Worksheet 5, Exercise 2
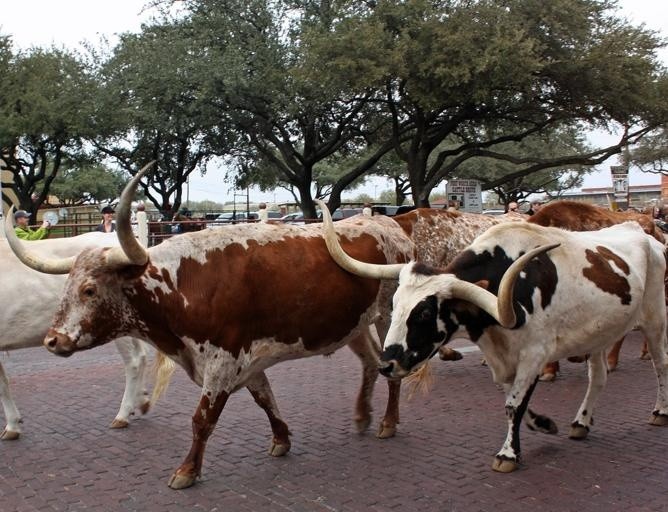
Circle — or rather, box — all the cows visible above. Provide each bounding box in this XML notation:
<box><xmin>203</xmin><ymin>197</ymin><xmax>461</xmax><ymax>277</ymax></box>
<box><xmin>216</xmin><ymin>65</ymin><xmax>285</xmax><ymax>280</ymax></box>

<box><xmin>0</xmin><ymin>231</ymin><xmax>179</xmax><ymax>441</ymax></box>
<box><xmin>311</xmin><ymin>198</ymin><xmax>668</xmax><ymax>474</ymax></box>
<box><xmin>390</xmin><ymin>207</ymin><xmax>530</xmax><ymax>361</ymax></box>
<box><xmin>527</xmin><ymin>201</ymin><xmax>665</xmax><ymax>382</ymax></box>
<box><xmin>5</xmin><ymin>160</ymin><xmax>432</xmax><ymax>490</ymax></box>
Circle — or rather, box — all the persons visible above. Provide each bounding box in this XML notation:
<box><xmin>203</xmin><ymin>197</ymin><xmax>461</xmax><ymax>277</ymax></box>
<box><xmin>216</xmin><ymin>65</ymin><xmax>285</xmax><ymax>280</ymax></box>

<box><xmin>507</xmin><ymin>201</ymin><xmax>519</xmax><ymax>213</ymax></box>
<box><xmin>653</xmin><ymin>204</ymin><xmax>668</xmax><ymax>235</ymax></box>
<box><xmin>93</xmin><ymin>206</ymin><xmax>117</xmax><ymax>233</ymax></box>
<box><xmin>524</xmin><ymin>201</ymin><xmax>541</xmax><ymax>216</ymax></box>
<box><xmin>13</xmin><ymin>210</ymin><xmax>51</xmax><ymax>241</ymax></box>
<box><xmin>173</xmin><ymin>207</ymin><xmax>190</xmax><ymax>226</ymax></box>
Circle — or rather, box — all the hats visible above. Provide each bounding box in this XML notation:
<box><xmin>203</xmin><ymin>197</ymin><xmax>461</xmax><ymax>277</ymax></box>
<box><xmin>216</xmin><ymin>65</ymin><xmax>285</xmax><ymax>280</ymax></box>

<box><xmin>531</xmin><ymin>199</ymin><xmax>544</xmax><ymax>205</ymax></box>
<box><xmin>101</xmin><ymin>207</ymin><xmax>115</xmax><ymax>214</ymax></box>
<box><xmin>510</xmin><ymin>202</ymin><xmax>517</xmax><ymax>209</ymax></box>
<box><xmin>14</xmin><ymin>210</ymin><xmax>32</xmax><ymax>218</ymax></box>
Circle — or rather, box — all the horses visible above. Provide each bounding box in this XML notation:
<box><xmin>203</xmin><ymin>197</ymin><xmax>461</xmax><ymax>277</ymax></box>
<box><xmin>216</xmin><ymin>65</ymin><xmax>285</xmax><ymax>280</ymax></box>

<box><xmin>170</xmin><ymin>212</ymin><xmax>206</xmax><ymax>233</ymax></box>
